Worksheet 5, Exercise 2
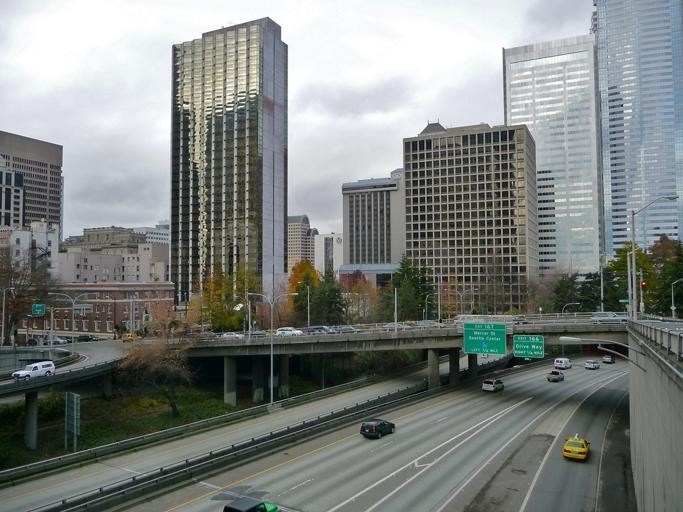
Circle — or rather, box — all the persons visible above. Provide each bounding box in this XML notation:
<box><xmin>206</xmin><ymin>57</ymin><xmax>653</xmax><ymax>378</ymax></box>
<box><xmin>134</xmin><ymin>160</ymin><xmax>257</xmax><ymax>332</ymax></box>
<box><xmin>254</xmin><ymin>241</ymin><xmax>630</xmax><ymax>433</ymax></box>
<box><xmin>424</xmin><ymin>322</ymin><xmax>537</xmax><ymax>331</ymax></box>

<box><xmin>439</xmin><ymin>318</ymin><xmax>442</xmax><ymax>323</ymax></box>
<box><xmin>113</xmin><ymin>332</ymin><xmax>116</xmax><ymax>340</ymax></box>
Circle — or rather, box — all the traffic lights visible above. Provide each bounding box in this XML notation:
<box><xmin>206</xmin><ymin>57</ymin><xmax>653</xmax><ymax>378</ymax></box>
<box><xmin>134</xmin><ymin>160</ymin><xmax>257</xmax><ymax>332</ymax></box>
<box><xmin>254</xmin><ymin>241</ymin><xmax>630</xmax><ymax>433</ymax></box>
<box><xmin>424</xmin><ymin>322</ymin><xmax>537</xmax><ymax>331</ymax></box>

<box><xmin>641</xmin><ymin>280</ymin><xmax>645</xmax><ymax>290</ymax></box>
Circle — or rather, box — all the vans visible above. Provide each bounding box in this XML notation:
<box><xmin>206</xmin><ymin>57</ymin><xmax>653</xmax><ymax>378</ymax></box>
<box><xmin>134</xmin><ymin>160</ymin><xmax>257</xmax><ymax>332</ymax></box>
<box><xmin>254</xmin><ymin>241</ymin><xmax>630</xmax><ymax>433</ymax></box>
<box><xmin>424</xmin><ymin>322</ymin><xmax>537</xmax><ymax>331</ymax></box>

<box><xmin>554</xmin><ymin>358</ymin><xmax>571</xmax><ymax>370</ymax></box>
<box><xmin>11</xmin><ymin>361</ymin><xmax>55</xmax><ymax>382</ymax></box>
<box><xmin>584</xmin><ymin>360</ymin><xmax>599</xmax><ymax>370</ymax></box>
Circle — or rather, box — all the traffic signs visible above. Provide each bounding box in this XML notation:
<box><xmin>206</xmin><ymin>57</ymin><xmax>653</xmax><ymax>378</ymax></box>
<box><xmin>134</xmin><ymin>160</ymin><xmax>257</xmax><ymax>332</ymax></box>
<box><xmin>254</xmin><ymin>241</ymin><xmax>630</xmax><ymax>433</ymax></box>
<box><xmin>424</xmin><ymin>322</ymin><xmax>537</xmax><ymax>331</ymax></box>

<box><xmin>31</xmin><ymin>304</ymin><xmax>45</xmax><ymax>315</ymax></box>
<box><xmin>618</xmin><ymin>299</ymin><xmax>628</xmax><ymax>303</ymax></box>
<box><xmin>462</xmin><ymin>322</ymin><xmax>506</xmax><ymax>356</ymax></box>
<box><xmin>512</xmin><ymin>335</ymin><xmax>544</xmax><ymax>359</ymax></box>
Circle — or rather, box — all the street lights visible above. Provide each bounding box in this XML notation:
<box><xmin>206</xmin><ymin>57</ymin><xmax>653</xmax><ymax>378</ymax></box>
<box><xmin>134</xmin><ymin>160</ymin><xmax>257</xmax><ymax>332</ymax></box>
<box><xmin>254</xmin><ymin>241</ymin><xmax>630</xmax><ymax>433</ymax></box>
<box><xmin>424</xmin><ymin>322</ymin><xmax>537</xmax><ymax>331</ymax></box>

<box><xmin>425</xmin><ymin>293</ymin><xmax>437</xmax><ymax>320</ymax></box>
<box><xmin>443</xmin><ymin>288</ymin><xmax>478</xmax><ymax>315</ymax></box>
<box><xmin>670</xmin><ymin>278</ymin><xmax>682</xmax><ymax>318</ymax></box>
<box><xmin>247</xmin><ymin>292</ymin><xmax>298</xmax><ymax>406</ymax></box>
<box><xmin>297</xmin><ymin>281</ymin><xmax>310</xmax><ymax>328</ymax></box>
<box><xmin>561</xmin><ymin>302</ymin><xmax>580</xmax><ymax>317</ymax></box>
<box><xmin>0</xmin><ymin>287</ymin><xmax>15</xmax><ymax>345</ymax></box>
<box><xmin>558</xmin><ymin>336</ymin><xmax>646</xmax><ymax>357</ymax></box>
<box><xmin>431</xmin><ymin>267</ymin><xmax>440</xmax><ymax>324</ymax></box>
<box><xmin>631</xmin><ymin>194</ymin><xmax>679</xmax><ymax>320</ymax></box>
<box><xmin>47</xmin><ymin>292</ymin><xmax>98</xmax><ymax>355</ymax></box>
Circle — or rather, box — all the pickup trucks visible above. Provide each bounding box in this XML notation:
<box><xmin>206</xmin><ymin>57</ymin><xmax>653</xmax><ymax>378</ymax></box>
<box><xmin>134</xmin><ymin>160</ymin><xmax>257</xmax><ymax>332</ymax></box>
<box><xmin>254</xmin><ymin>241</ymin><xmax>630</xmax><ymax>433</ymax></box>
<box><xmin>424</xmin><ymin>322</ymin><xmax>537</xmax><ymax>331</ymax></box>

<box><xmin>546</xmin><ymin>370</ymin><xmax>563</xmax><ymax>382</ymax></box>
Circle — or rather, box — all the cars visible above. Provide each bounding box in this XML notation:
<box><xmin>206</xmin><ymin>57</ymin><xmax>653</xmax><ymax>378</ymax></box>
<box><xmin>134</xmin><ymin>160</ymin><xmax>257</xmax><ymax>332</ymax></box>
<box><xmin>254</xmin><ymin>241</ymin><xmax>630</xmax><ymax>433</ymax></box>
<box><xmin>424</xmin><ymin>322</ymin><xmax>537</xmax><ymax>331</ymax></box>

<box><xmin>602</xmin><ymin>355</ymin><xmax>614</xmax><ymax>364</ymax></box>
<box><xmin>592</xmin><ymin>311</ymin><xmax>628</xmax><ymax>324</ymax></box>
<box><xmin>481</xmin><ymin>379</ymin><xmax>503</xmax><ymax>391</ymax></box>
<box><xmin>359</xmin><ymin>419</ymin><xmax>394</xmax><ymax>439</ymax></box>
<box><xmin>2</xmin><ymin>333</ymin><xmax>144</xmax><ymax>348</ymax></box>
<box><xmin>512</xmin><ymin>316</ymin><xmax>528</xmax><ymax>325</ymax></box>
<box><xmin>183</xmin><ymin>320</ymin><xmax>447</xmax><ymax>341</ymax></box>
<box><xmin>562</xmin><ymin>437</ymin><xmax>589</xmax><ymax>460</ymax></box>
